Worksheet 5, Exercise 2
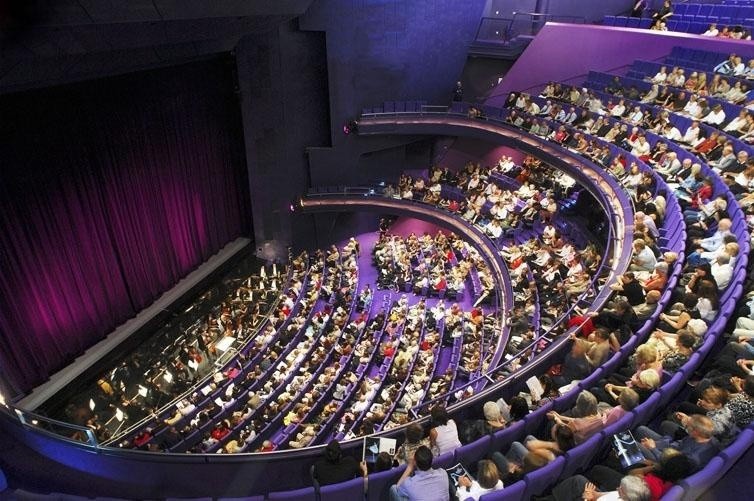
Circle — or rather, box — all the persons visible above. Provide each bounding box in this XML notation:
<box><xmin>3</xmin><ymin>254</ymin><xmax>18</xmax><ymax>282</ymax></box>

<box><xmin>651</xmin><ymin>1</ymin><xmax>673</xmax><ymax>21</ymax></box>
<box><xmin>60</xmin><ymin>53</ymin><xmax>753</xmax><ymax>501</ymax></box>
<box><xmin>701</xmin><ymin>23</ymin><xmax>752</xmax><ymax>40</ymax></box>
<box><xmin>651</xmin><ymin>21</ymin><xmax>669</xmax><ymax>31</ymax></box>
<box><xmin>629</xmin><ymin>0</ymin><xmax>648</xmax><ymax>18</ymax></box>
